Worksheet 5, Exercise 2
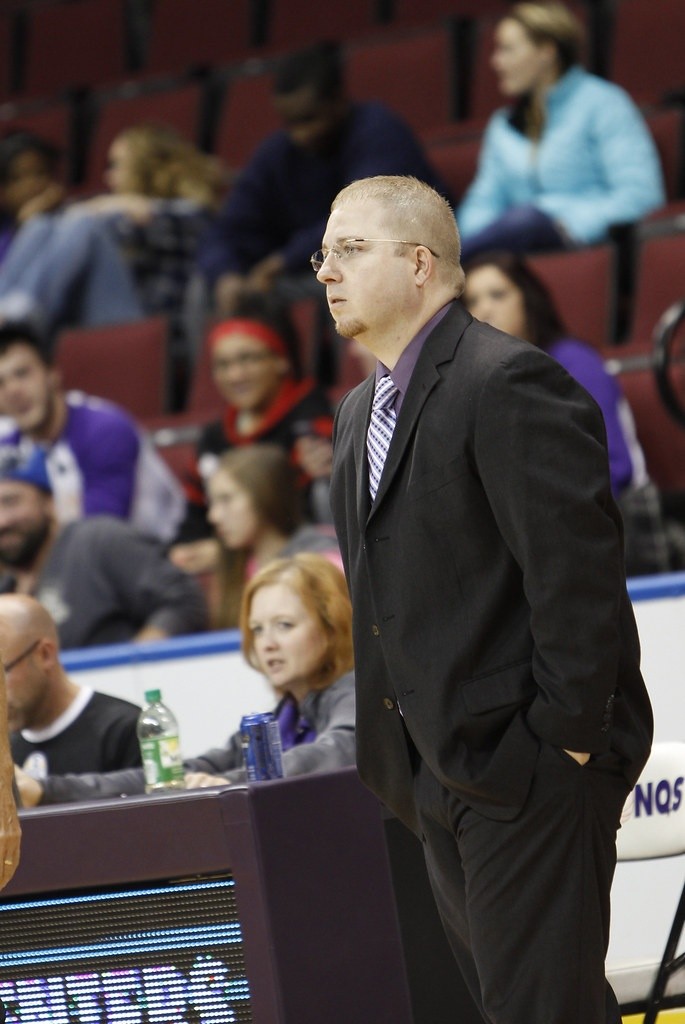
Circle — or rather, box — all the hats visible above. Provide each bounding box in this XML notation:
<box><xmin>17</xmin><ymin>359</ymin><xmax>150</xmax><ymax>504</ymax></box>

<box><xmin>0</xmin><ymin>443</ymin><xmax>53</xmax><ymax>495</ymax></box>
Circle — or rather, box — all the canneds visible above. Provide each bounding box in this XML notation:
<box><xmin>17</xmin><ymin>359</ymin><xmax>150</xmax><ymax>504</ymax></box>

<box><xmin>239</xmin><ymin>712</ymin><xmax>283</xmax><ymax>782</ymax></box>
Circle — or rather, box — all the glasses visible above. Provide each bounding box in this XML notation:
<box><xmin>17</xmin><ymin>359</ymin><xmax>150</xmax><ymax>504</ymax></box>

<box><xmin>310</xmin><ymin>238</ymin><xmax>439</xmax><ymax>272</ymax></box>
<box><xmin>279</xmin><ymin>103</ymin><xmax>329</xmax><ymax>130</ymax></box>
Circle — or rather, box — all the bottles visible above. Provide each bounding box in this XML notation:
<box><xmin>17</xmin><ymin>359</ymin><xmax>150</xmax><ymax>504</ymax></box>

<box><xmin>136</xmin><ymin>689</ymin><xmax>186</xmax><ymax>795</ymax></box>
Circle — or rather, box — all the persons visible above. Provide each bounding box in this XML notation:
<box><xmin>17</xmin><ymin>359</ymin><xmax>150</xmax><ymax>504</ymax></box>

<box><xmin>12</xmin><ymin>554</ymin><xmax>357</xmax><ymax>810</ymax></box>
<box><xmin>0</xmin><ymin>0</ymin><xmax>666</xmax><ymax>643</ymax></box>
<box><xmin>312</xmin><ymin>176</ymin><xmax>654</xmax><ymax>1024</ymax></box>
<box><xmin>0</xmin><ymin>589</ymin><xmax>160</xmax><ymax>808</ymax></box>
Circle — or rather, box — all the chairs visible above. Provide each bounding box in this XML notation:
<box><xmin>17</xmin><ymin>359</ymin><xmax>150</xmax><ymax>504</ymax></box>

<box><xmin>0</xmin><ymin>0</ymin><xmax>685</xmax><ymax>487</ymax></box>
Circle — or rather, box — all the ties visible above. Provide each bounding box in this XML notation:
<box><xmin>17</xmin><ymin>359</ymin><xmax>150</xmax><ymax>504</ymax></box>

<box><xmin>365</xmin><ymin>374</ymin><xmax>399</xmax><ymax>502</ymax></box>
